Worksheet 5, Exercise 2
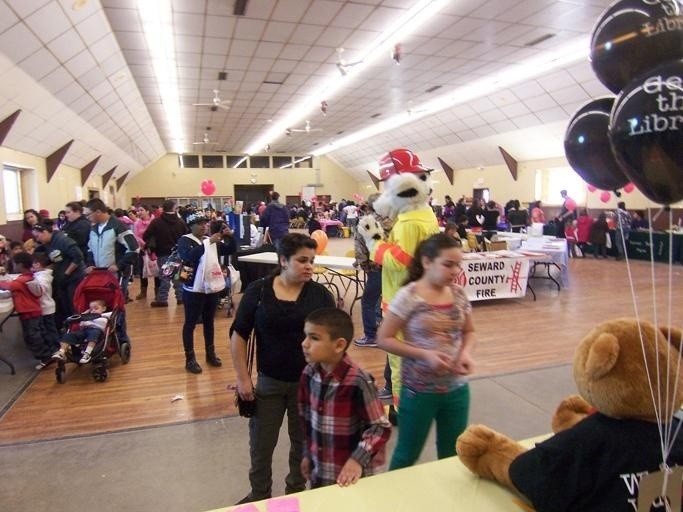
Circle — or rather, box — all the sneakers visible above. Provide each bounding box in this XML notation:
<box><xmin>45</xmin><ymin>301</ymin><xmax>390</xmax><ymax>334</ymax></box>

<box><xmin>567</xmin><ymin>252</ymin><xmax>625</xmax><ymax>262</ymax></box>
<box><xmin>352</xmin><ymin>332</ymin><xmax>380</xmax><ymax>348</ymax></box>
<box><xmin>376</xmin><ymin>386</ymin><xmax>394</xmax><ymax>401</ymax></box>
<box><xmin>34</xmin><ymin>360</ymin><xmax>51</xmax><ymax>370</ymax></box>
<box><xmin>77</xmin><ymin>352</ymin><xmax>92</xmax><ymax>364</ymax></box>
<box><xmin>150</xmin><ymin>298</ymin><xmax>185</xmax><ymax>308</ymax></box>
<box><xmin>51</xmin><ymin>350</ymin><xmax>68</xmax><ymax>362</ymax></box>
<box><xmin>233</xmin><ymin>494</ymin><xmax>274</xmax><ymax>507</ymax></box>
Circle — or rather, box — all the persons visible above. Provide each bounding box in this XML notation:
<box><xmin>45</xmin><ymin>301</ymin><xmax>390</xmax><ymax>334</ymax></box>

<box><xmin>229</xmin><ymin>234</ymin><xmax>336</xmax><ymax>506</ymax></box>
<box><xmin>429</xmin><ymin>189</ymin><xmax>651</xmax><ymax>260</ymax></box>
<box><xmin>296</xmin><ymin>306</ymin><xmax>392</xmax><ymax>490</ymax></box>
<box><xmin>375</xmin><ymin>233</ymin><xmax>475</xmax><ymax>472</ymax></box>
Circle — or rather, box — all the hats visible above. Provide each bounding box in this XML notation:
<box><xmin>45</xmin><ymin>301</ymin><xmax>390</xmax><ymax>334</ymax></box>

<box><xmin>184</xmin><ymin>206</ymin><xmax>211</xmax><ymax>225</ymax></box>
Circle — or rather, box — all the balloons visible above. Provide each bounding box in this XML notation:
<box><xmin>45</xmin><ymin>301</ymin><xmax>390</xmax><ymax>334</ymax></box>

<box><xmin>564</xmin><ymin>98</ymin><xmax>631</xmax><ymax>198</ymax></box>
<box><xmin>201</xmin><ymin>179</ymin><xmax>216</xmax><ymax>196</ymax></box>
<box><xmin>623</xmin><ymin>183</ymin><xmax>634</xmax><ymax>193</ymax></box>
<box><xmin>590</xmin><ymin>0</ymin><xmax>683</xmax><ymax>96</ymax></box>
<box><xmin>607</xmin><ymin>61</ymin><xmax>683</xmax><ymax>212</ymax></box>
<box><xmin>588</xmin><ymin>183</ymin><xmax>597</xmax><ymax>193</ymax></box>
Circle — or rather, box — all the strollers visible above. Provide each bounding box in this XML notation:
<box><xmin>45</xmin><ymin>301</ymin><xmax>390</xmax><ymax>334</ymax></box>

<box><xmin>53</xmin><ymin>265</ymin><xmax>131</xmax><ymax>388</ymax></box>
<box><xmin>213</xmin><ymin>254</ymin><xmax>236</xmax><ymax>318</ymax></box>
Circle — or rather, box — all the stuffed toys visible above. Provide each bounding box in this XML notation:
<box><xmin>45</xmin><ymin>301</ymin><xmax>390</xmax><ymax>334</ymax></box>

<box><xmin>455</xmin><ymin>317</ymin><xmax>683</xmax><ymax>512</ymax></box>
<box><xmin>355</xmin><ymin>172</ymin><xmax>439</xmax><ymax>425</ymax></box>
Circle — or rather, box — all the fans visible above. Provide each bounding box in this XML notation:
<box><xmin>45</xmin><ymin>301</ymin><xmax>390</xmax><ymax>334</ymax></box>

<box><xmin>193</xmin><ymin>48</ymin><xmax>427</xmax><ymax>145</ymax></box>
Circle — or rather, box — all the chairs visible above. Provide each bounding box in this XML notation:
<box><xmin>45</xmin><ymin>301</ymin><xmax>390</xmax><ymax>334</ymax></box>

<box><xmin>291</xmin><ymin>218</ymin><xmax>343</xmax><ymax>238</ymax></box>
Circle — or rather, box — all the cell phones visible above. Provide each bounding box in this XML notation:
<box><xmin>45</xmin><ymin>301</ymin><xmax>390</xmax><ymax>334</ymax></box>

<box><xmin>219</xmin><ymin>225</ymin><xmax>225</xmax><ymax>233</ymax></box>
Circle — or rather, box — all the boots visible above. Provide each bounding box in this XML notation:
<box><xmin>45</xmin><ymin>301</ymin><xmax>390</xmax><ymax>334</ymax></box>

<box><xmin>183</xmin><ymin>350</ymin><xmax>203</xmax><ymax>375</ymax></box>
<box><xmin>136</xmin><ymin>285</ymin><xmax>147</xmax><ymax>300</ymax></box>
<box><xmin>203</xmin><ymin>344</ymin><xmax>223</xmax><ymax>367</ymax></box>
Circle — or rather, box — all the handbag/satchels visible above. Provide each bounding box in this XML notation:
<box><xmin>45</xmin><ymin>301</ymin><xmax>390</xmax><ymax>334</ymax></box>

<box><xmin>234</xmin><ymin>385</ymin><xmax>257</xmax><ymax>420</ymax></box>
<box><xmin>159</xmin><ymin>235</ymin><xmax>196</xmax><ymax>283</ymax></box>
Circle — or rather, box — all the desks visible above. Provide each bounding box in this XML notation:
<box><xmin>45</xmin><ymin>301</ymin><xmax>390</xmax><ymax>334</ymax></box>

<box><xmin>438</xmin><ymin>219</ymin><xmax>569</xmax><ymax>302</ymax></box>
<box><xmin>237</xmin><ymin>251</ymin><xmax>368</xmax><ymax>316</ymax></box>
<box><xmin>205</xmin><ymin>432</ymin><xmax>555</xmax><ymax>512</ymax></box>
<box><xmin>0</xmin><ymin>272</ymin><xmax>32</xmax><ymax>376</ymax></box>
